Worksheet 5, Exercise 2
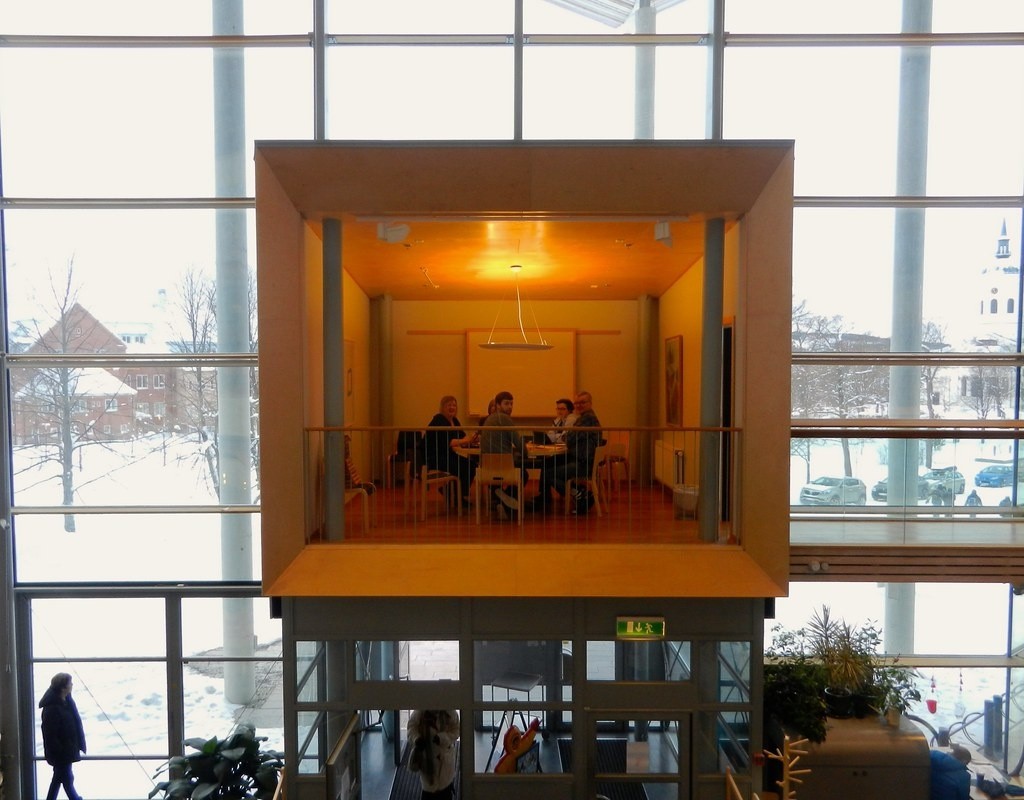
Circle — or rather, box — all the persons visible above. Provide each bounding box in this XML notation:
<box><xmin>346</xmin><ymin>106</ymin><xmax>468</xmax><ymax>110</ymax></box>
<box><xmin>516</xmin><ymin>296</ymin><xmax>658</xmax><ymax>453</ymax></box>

<box><xmin>420</xmin><ymin>396</ymin><xmax>480</xmax><ymax>510</ymax></box>
<box><xmin>38</xmin><ymin>673</ymin><xmax>86</xmax><ymax>800</ymax></box>
<box><xmin>932</xmin><ymin>486</ymin><xmax>1013</xmax><ymax>518</ymax></box>
<box><xmin>930</xmin><ymin>747</ymin><xmax>974</xmax><ymax>800</ymax></box>
<box><xmin>407</xmin><ymin>710</ymin><xmax>460</xmax><ymax>800</ymax></box>
<box><xmin>533</xmin><ymin>391</ymin><xmax>602</xmax><ymax>515</ymax></box>
<box><xmin>479</xmin><ymin>392</ymin><xmax>529</xmax><ymax>520</ymax></box>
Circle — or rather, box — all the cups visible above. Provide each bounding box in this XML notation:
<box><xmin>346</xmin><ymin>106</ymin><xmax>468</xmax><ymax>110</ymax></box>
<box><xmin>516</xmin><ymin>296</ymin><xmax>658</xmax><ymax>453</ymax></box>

<box><xmin>977</xmin><ymin>773</ymin><xmax>984</xmax><ymax>785</ymax></box>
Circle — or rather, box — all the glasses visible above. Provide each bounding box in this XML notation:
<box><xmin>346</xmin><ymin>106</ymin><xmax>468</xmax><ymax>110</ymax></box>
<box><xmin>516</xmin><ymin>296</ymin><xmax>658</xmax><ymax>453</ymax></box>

<box><xmin>574</xmin><ymin>400</ymin><xmax>590</xmax><ymax>404</ymax></box>
<box><xmin>556</xmin><ymin>408</ymin><xmax>567</xmax><ymax>411</ymax></box>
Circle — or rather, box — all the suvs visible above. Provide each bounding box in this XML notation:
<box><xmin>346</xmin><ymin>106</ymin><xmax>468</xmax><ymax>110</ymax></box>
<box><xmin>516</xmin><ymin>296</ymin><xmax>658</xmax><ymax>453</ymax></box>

<box><xmin>800</xmin><ymin>476</ymin><xmax>868</xmax><ymax>506</ymax></box>
<box><xmin>921</xmin><ymin>466</ymin><xmax>965</xmax><ymax>495</ymax></box>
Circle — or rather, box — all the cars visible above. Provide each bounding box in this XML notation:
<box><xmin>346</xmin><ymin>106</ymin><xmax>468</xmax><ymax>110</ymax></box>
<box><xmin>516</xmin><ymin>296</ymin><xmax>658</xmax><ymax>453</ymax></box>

<box><xmin>872</xmin><ymin>475</ymin><xmax>930</xmax><ymax>502</ymax></box>
<box><xmin>974</xmin><ymin>465</ymin><xmax>1014</xmax><ymax>488</ymax></box>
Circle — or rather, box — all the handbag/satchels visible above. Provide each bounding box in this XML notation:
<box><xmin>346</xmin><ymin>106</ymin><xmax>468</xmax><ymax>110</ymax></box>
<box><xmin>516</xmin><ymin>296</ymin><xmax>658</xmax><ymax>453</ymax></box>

<box><xmin>408</xmin><ymin>746</ymin><xmax>421</xmax><ymax>773</ymax></box>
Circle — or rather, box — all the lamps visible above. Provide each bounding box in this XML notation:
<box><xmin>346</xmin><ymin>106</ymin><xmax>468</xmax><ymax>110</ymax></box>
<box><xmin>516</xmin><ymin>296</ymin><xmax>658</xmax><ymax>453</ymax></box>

<box><xmin>654</xmin><ymin>223</ymin><xmax>674</xmax><ymax>248</ymax></box>
<box><xmin>376</xmin><ymin>220</ymin><xmax>408</xmax><ymax>243</ymax></box>
<box><xmin>478</xmin><ymin>264</ymin><xmax>554</xmax><ymax>351</ymax></box>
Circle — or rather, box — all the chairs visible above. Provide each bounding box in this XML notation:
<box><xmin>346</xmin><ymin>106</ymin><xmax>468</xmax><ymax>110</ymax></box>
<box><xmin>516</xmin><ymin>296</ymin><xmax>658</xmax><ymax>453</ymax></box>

<box><xmin>318</xmin><ymin>436</ymin><xmax>377</xmax><ymax>533</ymax></box>
<box><xmin>386</xmin><ymin>430</ymin><xmax>423</xmax><ymax>495</ymax></box>
<box><xmin>405</xmin><ymin>440</ymin><xmax>462</xmax><ymax>522</ymax></box>
<box><xmin>597</xmin><ymin>431</ymin><xmax>630</xmax><ymax>493</ymax></box>
<box><xmin>566</xmin><ymin>441</ymin><xmax>611</xmax><ymax>517</ymax></box>
<box><xmin>474</xmin><ymin>454</ymin><xmax>524</xmax><ymax>525</ymax></box>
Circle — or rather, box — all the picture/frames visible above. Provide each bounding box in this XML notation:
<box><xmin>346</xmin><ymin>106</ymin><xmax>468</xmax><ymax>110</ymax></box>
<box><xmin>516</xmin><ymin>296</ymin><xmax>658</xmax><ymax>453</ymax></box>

<box><xmin>663</xmin><ymin>335</ymin><xmax>682</xmax><ymax>425</ymax></box>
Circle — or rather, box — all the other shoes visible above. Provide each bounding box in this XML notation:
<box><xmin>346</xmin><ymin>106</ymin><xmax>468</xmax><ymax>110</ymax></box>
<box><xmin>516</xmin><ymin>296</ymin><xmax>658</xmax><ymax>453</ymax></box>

<box><xmin>494</xmin><ymin>488</ymin><xmax>518</xmax><ymax>520</ymax></box>
<box><xmin>575</xmin><ymin>483</ymin><xmax>595</xmax><ymax>515</ymax></box>
<box><xmin>530</xmin><ymin>493</ymin><xmax>553</xmax><ymax>513</ymax></box>
<box><xmin>438</xmin><ymin>484</ymin><xmax>472</xmax><ymax>509</ymax></box>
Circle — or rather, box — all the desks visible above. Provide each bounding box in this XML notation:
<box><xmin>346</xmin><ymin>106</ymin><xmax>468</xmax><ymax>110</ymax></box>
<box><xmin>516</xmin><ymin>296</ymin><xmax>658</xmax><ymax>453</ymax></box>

<box><xmin>490</xmin><ymin>672</ymin><xmax>544</xmax><ymax>746</ymax></box>
<box><xmin>787</xmin><ymin>710</ymin><xmax>930</xmax><ymax>800</ymax></box>
<box><xmin>451</xmin><ymin>439</ymin><xmax>569</xmax><ymax>459</ymax></box>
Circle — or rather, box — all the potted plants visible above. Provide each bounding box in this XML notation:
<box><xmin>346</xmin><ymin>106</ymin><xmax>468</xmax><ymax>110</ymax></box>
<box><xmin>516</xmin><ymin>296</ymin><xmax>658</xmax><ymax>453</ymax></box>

<box><xmin>768</xmin><ymin>610</ymin><xmax>920</xmax><ymax>730</ymax></box>
